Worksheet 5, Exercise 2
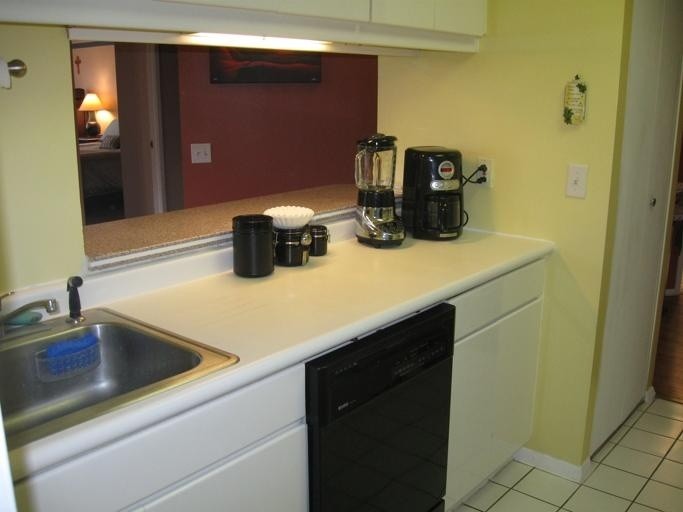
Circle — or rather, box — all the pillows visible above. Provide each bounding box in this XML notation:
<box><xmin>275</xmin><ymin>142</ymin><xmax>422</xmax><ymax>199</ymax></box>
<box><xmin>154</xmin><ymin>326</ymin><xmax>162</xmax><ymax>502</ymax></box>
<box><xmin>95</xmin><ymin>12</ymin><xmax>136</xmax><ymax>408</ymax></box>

<box><xmin>97</xmin><ymin>112</ymin><xmax>120</xmax><ymax>150</ymax></box>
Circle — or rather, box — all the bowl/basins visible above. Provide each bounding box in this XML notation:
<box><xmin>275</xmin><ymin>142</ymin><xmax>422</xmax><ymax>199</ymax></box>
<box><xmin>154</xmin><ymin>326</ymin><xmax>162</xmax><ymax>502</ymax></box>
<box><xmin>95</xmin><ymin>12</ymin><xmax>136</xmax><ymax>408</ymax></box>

<box><xmin>263</xmin><ymin>205</ymin><xmax>314</xmax><ymax>230</ymax></box>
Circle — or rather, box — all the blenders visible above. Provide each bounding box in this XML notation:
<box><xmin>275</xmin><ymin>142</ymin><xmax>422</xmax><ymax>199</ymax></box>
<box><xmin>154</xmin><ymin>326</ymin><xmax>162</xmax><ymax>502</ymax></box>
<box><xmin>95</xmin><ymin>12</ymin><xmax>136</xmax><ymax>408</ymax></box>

<box><xmin>354</xmin><ymin>133</ymin><xmax>405</xmax><ymax>247</ymax></box>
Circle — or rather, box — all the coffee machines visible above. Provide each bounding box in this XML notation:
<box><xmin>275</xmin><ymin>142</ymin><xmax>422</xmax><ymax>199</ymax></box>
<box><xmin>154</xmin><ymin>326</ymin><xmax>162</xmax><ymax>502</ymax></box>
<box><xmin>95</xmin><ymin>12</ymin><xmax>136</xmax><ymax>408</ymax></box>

<box><xmin>401</xmin><ymin>145</ymin><xmax>465</xmax><ymax>241</ymax></box>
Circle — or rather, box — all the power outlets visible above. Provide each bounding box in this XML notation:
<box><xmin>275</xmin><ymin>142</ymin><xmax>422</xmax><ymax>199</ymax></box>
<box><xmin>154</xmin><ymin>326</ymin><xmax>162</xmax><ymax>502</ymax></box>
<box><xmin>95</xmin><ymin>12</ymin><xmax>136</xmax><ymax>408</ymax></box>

<box><xmin>473</xmin><ymin>159</ymin><xmax>494</xmax><ymax>190</ymax></box>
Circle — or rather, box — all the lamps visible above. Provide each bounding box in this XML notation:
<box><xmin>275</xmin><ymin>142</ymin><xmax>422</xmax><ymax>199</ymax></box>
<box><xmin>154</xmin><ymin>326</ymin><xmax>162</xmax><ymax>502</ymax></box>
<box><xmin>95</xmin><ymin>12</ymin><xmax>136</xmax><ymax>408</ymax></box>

<box><xmin>78</xmin><ymin>94</ymin><xmax>101</xmax><ymax>139</ymax></box>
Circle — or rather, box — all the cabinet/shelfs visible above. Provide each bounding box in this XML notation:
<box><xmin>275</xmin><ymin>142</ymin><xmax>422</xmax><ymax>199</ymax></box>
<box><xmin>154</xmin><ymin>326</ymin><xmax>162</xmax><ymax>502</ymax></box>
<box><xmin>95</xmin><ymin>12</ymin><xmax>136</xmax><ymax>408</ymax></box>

<box><xmin>171</xmin><ymin>1</ymin><xmax>370</xmax><ymax>54</ymax></box>
<box><xmin>5</xmin><ymin>363</ymin><xmax>310</xmax><ymax>512</ymax></box>
<box><xmin>370</xmin><ymin>1</ymin><xmax>488</xmax><ymax>54</ymax></box>
<box><xmin>444</xmin><ymin>241</ymin><xmax>555</xmax><ymax>512</ymax></box>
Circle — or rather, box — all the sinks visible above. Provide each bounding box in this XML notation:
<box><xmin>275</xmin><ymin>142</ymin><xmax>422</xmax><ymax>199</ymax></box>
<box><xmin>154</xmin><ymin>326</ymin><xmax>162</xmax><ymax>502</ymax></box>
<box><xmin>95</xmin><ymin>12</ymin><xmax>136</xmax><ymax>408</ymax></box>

<box><xmin>3</xmin><ymin>306</ymin><xmax>240</xmax><ymax>452</ymax></box>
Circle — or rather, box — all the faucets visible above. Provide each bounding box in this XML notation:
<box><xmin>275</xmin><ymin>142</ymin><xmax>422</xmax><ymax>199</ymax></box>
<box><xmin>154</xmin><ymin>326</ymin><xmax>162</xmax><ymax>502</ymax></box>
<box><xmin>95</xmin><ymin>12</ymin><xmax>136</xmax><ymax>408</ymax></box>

<box><xmin>1</xmin><ymin>290</ymin><xmax>58</xmax><ymax>338</ymax></box>
